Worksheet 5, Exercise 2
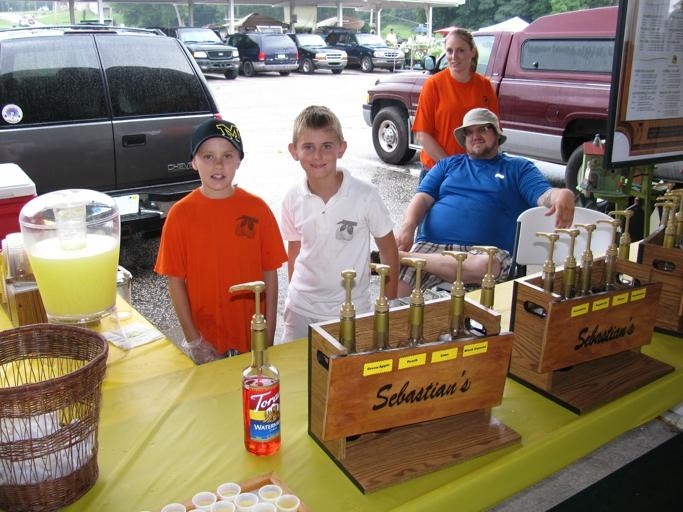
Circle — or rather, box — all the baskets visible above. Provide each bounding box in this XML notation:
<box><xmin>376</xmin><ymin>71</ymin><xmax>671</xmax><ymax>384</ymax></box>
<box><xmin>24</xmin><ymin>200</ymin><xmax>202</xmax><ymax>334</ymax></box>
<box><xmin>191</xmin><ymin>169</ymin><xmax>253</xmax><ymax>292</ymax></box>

<box><xmin>0</xmin><ymin>323</ymin><xmax>109</xmax><ymax>511</ymax></box>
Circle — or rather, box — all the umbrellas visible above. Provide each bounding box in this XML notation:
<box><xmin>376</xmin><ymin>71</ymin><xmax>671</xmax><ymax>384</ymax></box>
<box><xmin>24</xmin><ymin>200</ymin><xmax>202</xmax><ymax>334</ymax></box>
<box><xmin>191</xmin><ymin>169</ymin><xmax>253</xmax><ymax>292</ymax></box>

<box><xmin>409</xmin><ymin>26</ymin><xmax>428</xmax><ymax>32</ymax></box>
<box><xmin>434</xmin><ymin>27</ymin><xmax>459</xmax><ymax>34</ymax></box>
<box><xmin>477</xmin><ymin>16</ymin><xmax>531</xmax><ymax>32</ymax></box>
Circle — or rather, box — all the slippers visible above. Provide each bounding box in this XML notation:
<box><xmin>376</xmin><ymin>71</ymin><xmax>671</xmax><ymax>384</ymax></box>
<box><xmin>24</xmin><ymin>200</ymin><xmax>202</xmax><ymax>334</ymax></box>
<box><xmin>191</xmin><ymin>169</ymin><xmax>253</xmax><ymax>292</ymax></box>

<box><xmin>371</xmin><ymin>251</ymin><xmax>381</xmax><ymax>274</ymax></box>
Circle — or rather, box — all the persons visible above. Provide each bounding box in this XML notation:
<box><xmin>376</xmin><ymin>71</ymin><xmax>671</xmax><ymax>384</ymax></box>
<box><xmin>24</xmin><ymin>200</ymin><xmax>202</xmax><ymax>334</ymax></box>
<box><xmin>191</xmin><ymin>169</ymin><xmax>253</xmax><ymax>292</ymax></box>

<box><xmin>153</xmin><ymin>118</ymin><xmax>290</xmax><ymax>366</ymax></box>
<box><xmin>282</xmin><ymin>105</ymin><xmax>400</xmax><ymax>344</ymax></box>
<box><xmin>371</xmin><ymin>107</ymin><xmax>575</xmax><ymax>308</ymax></box>
<box><xmin>385</xmin><ymin>27</ymin><xmax>400</xmax><ymax>49</ymax></box>
<box><xmin>398</xmin><ymin>32</ymin><xmax>434</xmax><ymax>54</ymax></box>
<box><xmin>419</xmin><ymin>30</ymin><xmax>503</xmax><ymax>195</ymax></box>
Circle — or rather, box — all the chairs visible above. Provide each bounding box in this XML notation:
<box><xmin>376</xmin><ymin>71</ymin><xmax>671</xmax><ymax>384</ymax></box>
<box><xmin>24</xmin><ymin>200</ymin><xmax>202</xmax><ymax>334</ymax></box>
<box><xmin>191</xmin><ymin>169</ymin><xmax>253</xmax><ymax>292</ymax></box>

<box><xmin>506</xmin><ymin>205</ymin><xmax>621</xmax><ymax>280</ymax></box>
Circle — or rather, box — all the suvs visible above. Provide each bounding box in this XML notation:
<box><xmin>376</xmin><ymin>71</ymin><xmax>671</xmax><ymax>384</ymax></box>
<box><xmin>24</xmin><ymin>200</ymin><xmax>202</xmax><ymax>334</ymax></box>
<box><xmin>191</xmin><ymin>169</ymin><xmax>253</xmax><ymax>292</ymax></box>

<box><xmin>290</xmin><ymin>34</ymin><xmax>346</xmax><ymax>74</ymax></box>
<box><xmin>227</xmin><ymin>33</ymin><xmax>299</xmax><ymax>77</ymax></box>
<box><xmin>326</xmin><ymin>33</ymin><xmax>406</xmax><ymax>73</ymax></box>
<box><xmin>0</xmin><ymin>26</ymin><xmax>222</xmax><ymax>238</ymax></box>
<box><xmin>156</xmin><ymin>27</ymin><xmax>240</xmax><ymax>79</ymax></box>
<box><xmin>363</xmin><ymin>5</ymin><xmax>682</xmax><ymax>213</ymax></box>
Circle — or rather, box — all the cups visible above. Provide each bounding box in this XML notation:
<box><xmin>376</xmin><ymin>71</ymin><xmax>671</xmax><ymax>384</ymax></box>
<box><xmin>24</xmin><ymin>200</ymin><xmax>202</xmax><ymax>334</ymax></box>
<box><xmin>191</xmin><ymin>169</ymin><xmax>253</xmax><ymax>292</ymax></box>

<box><xmin>235</xmin><ymin>493</ymin><xmax>259</xmax><ymax>512</ymax></box>
<box><xmin>190</xmin><ymin>509</ymin><xmax>207</xmax><ymax>512</ymax></box>
<box><xmin>275</xmin><ymin>495</ymin><xmax>301</xmax><ymax>512</ymax></box>
<box><xmin>258</xmin><ymin>485</ymin><xmax>282</xmax><ymax>502</ymax></box>
<box><xmin>252</xmin><ymin>503</ymin><xmax>277</xmax><ymax>512</ymax></box>
<box><xmin>217</xmin><ymin>483</ymin><xmax>242</xmax><ymax>500</ymax></box>
<box><xmin>192</xmin><ymin>492</ymin><xmax>217</xmax><ymax>508</ymax></box>
<box><xmin>160</xmin><ymin>501</ymin><xmax>188</xmax><ymax>512</ymax></box>
<box><xmin>210</xmin><ymin>501</ymin><xmax>235</xmax><ymax>512</ymax></box>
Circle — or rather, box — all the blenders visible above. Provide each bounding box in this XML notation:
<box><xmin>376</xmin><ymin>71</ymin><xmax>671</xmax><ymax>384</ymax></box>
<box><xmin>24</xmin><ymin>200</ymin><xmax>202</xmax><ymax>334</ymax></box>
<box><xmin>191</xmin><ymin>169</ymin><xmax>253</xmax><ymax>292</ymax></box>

<box><xmin>19</xmin><ymin>189</ymin><xmax>131</xmax><ymax>366</ymax></box>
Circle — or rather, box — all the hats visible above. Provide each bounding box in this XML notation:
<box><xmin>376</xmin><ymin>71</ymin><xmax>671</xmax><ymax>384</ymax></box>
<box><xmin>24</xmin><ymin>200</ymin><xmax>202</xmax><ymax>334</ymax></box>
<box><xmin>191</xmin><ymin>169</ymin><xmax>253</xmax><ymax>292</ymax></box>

<box><xmin>192</xmin><ymin>119</ymin><xmax>244</xmax><ymax>160</ymax></box>
<box><xmin>454</xmin><ymin>108</ymin><xmax>506</xmax><ymax>148</ymax></box>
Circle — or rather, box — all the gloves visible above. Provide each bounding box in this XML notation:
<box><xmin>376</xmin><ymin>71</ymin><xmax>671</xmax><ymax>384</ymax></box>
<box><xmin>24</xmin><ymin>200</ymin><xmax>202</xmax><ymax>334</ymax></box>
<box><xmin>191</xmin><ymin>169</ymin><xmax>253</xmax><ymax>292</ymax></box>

<box><xmin>181</xmin><ymin>336</ymin><xmax>220</xmax><ymax>364</ymax></box>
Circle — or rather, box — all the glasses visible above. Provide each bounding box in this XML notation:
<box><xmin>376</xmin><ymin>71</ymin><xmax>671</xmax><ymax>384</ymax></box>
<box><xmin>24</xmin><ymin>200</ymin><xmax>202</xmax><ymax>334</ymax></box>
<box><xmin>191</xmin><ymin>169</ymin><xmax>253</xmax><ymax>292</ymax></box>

<box><xmin>462</xmin><ymin>125</ymin><xmax>494</xmax><ymax>136</ymax></box>
